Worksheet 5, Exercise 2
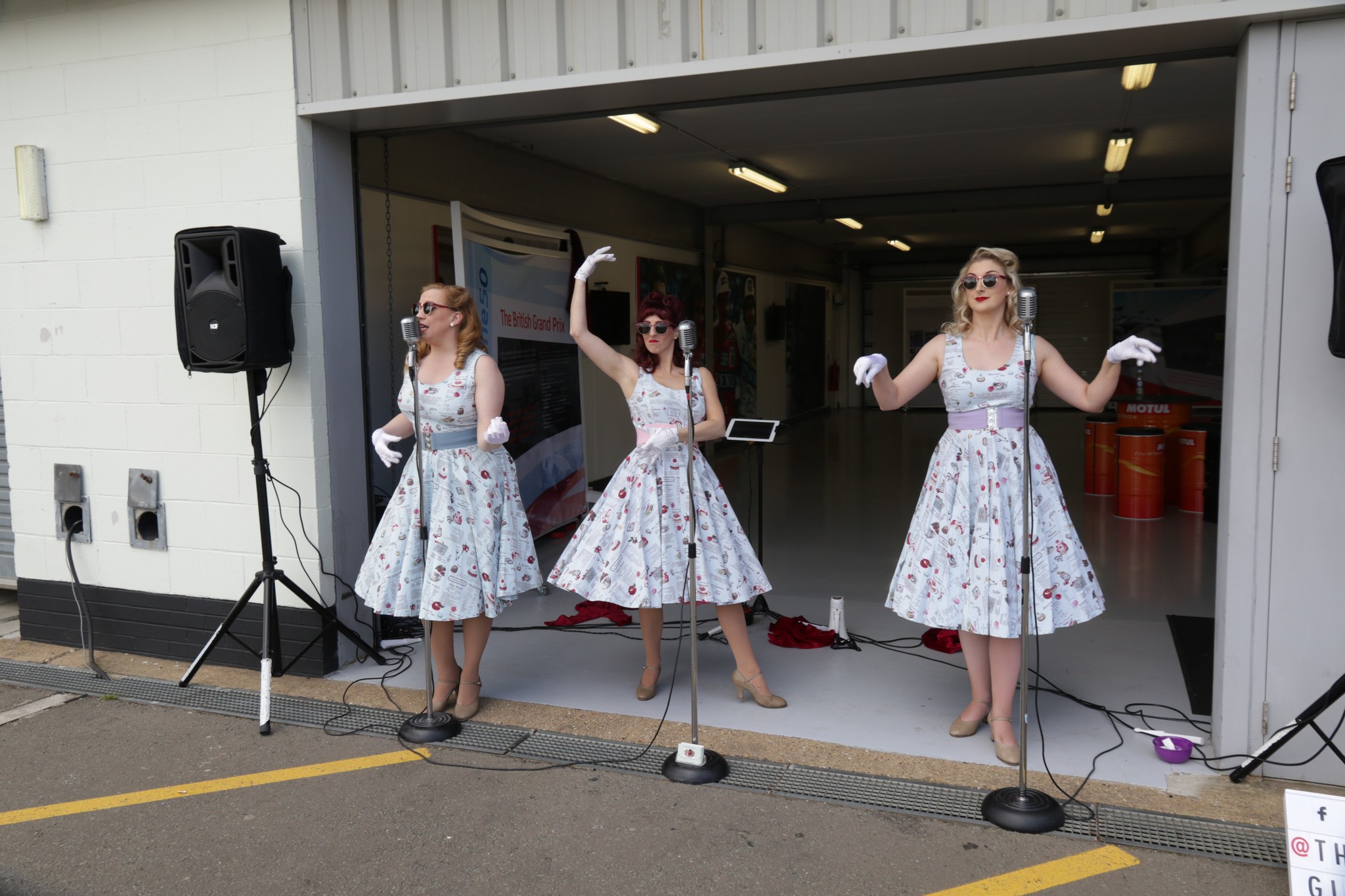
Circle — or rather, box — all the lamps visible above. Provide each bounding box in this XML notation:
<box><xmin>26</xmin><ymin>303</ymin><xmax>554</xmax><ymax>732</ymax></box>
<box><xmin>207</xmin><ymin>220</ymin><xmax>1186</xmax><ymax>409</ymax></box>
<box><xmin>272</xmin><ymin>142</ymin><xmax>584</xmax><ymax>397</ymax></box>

<box><xmin>16</xmin><ymin>145</ymin><xmax>50</xmax><ymax>223</ymax></box>
<box><xmin>728</xmin><ymin>161</ymin><xmax>790</xmax><ymax>193</ymax></box>
<box><xmin>606</xmin><ymin>113</ymin><xmax>661</xmax><ymax>135</ymax></box>
<box><xmin>1121</xmin><ymin>63</ymin><xmax>1157</xmax><ymax>90</ymax></box>
<box><xmin>1103</xmin><ymin>129</ymin><xmax>1134</xmax><ymax>172</ymax></box>
<box><xmin>835</xmin><ymin>218</ymin><xmax>911</xmax><ymax>252</ymax></box>
<box><xmin>1090</xmin><ymin>204</ymin><xmax>1113</xmax><ymax>244</ymax></box>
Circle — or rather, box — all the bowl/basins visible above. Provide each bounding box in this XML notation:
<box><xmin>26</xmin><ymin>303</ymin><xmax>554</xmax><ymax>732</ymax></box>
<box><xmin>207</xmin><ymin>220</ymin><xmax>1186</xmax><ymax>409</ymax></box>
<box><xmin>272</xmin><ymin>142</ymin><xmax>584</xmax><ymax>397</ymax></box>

<box><xmin>1152</xmin><ymin>735</ymin><xmax>1192</xmax><ymax>764</ymax></box>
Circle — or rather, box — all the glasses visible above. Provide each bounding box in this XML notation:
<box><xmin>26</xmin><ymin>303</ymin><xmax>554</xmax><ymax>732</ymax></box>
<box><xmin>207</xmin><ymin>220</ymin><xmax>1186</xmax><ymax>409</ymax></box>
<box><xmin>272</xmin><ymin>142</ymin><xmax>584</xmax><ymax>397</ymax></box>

<box><xmin>636</xmin><ymin>321</ymin><xmax>676</xmax><ymax>335</ymax></box>
<box><xmin>961</xmin><ymin>273</ymin><xmax>1008</xmax><ymax>290</ymax></box>
<box><xmin>412</xmin><ymin>302</ymin><xmax>459</xmax><ymax>315</ymax></box>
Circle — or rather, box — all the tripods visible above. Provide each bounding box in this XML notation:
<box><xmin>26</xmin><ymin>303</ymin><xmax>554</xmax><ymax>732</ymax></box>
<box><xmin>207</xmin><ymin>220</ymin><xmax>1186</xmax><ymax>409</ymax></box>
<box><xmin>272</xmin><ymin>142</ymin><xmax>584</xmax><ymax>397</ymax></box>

<box><xmin>176</xmin><ymin>370</ymin><xmax>385</xmax><ymax>736</ymax></box>
<box><xmin>698</xmin><ymin>443</ymin><xmax>789</xmax><ymax>640</ymax></box>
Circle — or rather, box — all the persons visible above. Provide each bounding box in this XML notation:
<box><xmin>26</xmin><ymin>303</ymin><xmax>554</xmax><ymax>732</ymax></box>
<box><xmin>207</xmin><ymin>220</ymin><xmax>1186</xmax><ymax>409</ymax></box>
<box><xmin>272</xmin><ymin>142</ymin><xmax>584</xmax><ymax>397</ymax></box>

<box><xmin>546</xmin><ymin>247</ymin><xmax>788</xmax><ymax>708</ymax></box>
<box><xmin>854</xmin><ymin>247</ymin><xmax>1161</xmax><ymax>767</ymax></box>
<box><xmin>353</xmin><ymin>285</ymin><xmax>543</xmax><ymax>722</ymax></box>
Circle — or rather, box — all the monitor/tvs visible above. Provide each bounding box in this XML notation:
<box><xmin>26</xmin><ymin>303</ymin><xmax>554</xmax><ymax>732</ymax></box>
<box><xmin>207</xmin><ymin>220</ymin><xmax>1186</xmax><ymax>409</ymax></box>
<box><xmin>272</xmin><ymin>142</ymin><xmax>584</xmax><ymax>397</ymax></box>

<box><xmin>590</xmin><ymin>290</ymin><xmax>631</xmax><ymax>346</ymax></box>
<box><xmin>764</xmin><ymin>305</ymin><xmax>786</xmax><ymax>341</ymax></box>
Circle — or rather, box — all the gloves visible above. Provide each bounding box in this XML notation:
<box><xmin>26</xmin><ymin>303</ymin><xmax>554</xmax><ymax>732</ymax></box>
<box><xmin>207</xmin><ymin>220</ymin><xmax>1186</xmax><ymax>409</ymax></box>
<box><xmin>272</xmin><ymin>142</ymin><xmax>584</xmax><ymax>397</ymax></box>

<box><xmin>636</xmin><ymin>425</ymin><xmax>679</xmax><ymax>466</ymax></box>
<box><xmin>574</xmin><ymin>246</ymin><xmax>616</xmax><ymax>282</ymax></box>
<box><xmin>484</xmin><ymin>416</ymin><xmax>510</xmax><ymax>444</ymax></box>
<box><xmin>372</xmin><ymin>429</ymin><xmax>402</xmax><ymax>468</ymax></box>
<box><xmin>1107</xmin><ymin>335</ymin><xmax>1162</xmax><ymax>366</ymax></box>
<box><xmin>853</xmin><ymin>353</ymin><xmax>887</xmax><ymax>388</ymax></box>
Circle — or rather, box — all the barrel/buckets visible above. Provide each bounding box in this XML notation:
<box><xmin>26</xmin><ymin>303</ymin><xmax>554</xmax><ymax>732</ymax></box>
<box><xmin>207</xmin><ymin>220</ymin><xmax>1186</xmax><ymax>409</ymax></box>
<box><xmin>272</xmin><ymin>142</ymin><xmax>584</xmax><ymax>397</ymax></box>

<box><xmin>1085</xmin><ymin>415</ymin><xmax>1117</xmax><ymax>497</ymax></box>
<box><xmin>1113</xmin><ymin>426</ymin><xmax>1164</xmax><ymax>521</ymax></box>
<box><xmin>1177</xmin><ymin>424</ymin><xmax>1208</xmax><ymax>514</ymax></box>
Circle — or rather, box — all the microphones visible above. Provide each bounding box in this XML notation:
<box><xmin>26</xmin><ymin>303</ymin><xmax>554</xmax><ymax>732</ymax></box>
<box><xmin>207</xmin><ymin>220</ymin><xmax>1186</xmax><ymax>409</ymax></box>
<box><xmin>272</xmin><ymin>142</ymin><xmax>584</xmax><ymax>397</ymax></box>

<box><xmin>676</xmin><ymin>320</ymin><xmax>697</xmax><ymax>350</ymax></box>
<box><xmin>1017</xmin><ymin>287</ymin><xmax>1037</xmax><ymax>321</ymax></box>
<box><xmin>400</xmin><ymin>316</ymin><xmax>421</xmax><ymax>343</ymax></box>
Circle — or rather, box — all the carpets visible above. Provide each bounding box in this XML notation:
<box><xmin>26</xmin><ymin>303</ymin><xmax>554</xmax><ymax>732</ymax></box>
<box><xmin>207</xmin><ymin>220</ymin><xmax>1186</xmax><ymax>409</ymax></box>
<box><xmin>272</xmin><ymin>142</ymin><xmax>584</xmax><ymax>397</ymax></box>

<box><xmin>1167</xmin><ymin>613</ymin><xmax>1214</xmax><ymax>716</ymax></box>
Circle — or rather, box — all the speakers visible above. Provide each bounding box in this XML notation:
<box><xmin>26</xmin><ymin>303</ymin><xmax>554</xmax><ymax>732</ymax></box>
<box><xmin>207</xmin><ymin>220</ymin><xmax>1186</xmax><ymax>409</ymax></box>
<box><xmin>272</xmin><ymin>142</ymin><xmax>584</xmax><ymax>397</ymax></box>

<box><xmin>1315</xmin><ymin>156</ymin><xmax>1345</xmax><ymax>359</ymax></box>
<box><xmin>174</xmin><ymin>225</ymin><xmax>294</xmax><ymax>374</ymax></box>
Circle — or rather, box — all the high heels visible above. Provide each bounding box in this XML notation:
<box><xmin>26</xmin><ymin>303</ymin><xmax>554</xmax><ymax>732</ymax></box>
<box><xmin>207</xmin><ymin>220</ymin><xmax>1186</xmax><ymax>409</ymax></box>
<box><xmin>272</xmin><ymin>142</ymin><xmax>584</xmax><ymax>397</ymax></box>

<box><xmin>731</xmin><ymin>669</ymin><xmax>787</xmax><ymax>709</ymax></box>
<box><xmin>454</xmin><ymin>675</ymin><xmax>482</xmax><ymax>721</ymax></box>
<box><xmin>432</xmin><ymin>666</ymin><xmax>462</xmax><ymax>713</ymax></box>
<box><xmin>950</xmin><ymin>697</ymin><xmax>992</xmax><ymax>737</ymax></box>
<box><xmin>637</xmin><ymin>667</ymin><xmax>662</xmax><ymax>701</ymax></box>
<box><xmin>988</xmin><ymin>709</ymin><xmax>1021</xmax><ymax>766</ymax></box>
